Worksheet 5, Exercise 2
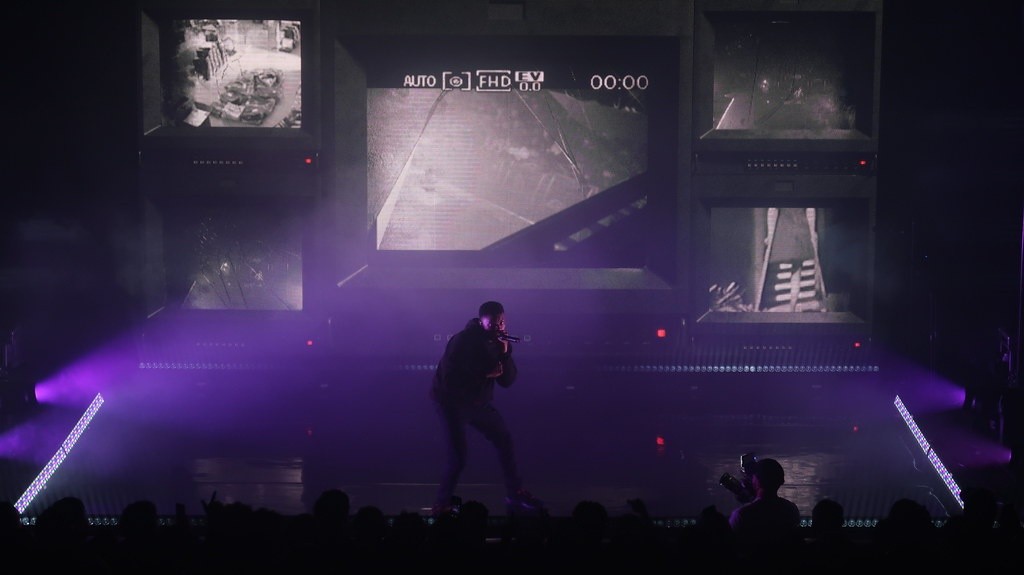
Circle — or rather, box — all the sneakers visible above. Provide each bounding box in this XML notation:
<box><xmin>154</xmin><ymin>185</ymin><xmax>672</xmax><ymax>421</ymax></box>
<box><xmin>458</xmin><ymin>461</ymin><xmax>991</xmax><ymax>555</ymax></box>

<box><xmin>502</xmin><ymin>487</ymin><xmax>538</xmax><ymax>511</ymax></box>
<box><xmin>442</xmin><ymin>501</ymin><xmax>463</xmax><ymax>519</ymax></box>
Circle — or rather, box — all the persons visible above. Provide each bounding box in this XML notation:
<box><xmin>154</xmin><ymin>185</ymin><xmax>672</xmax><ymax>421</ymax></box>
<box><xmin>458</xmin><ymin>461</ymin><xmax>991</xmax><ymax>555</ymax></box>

<box><xmin>0</xmin><ymin>458</ymin><xmax>1024</xmax><ymax>575</ymax></box>
<box><xmin>429</xmin><ymin>301</ymin><xmax>545</xmax><ymax>510</ymax></box>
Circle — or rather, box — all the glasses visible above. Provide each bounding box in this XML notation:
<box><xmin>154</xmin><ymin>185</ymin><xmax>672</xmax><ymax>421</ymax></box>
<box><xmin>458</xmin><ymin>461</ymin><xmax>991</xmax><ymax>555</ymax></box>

<box><xmin>482</xmin><ymin>321</ymin><xmax>505</xmax><ymax>327</ymax></box>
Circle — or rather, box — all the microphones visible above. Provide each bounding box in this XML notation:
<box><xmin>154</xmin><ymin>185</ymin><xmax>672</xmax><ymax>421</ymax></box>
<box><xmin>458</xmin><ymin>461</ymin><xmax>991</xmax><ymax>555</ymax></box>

<box><xmin>498</xmin><ymin>334</ymin><xmax>520</xmax><ymax>343</ymax></box>
